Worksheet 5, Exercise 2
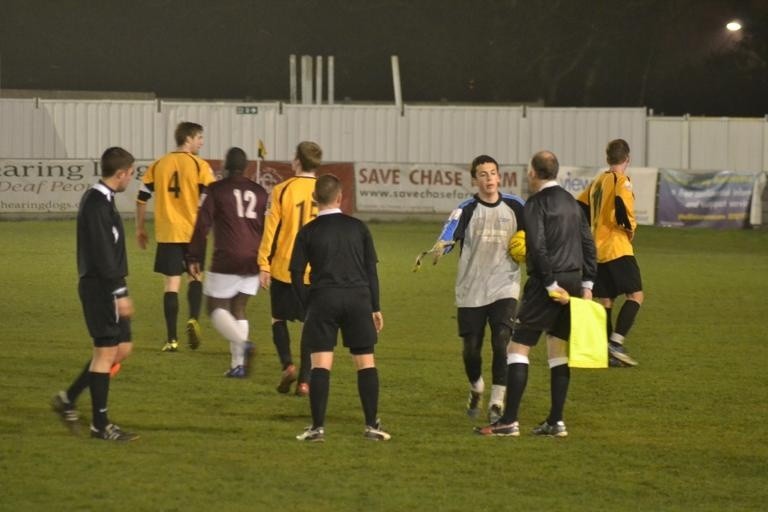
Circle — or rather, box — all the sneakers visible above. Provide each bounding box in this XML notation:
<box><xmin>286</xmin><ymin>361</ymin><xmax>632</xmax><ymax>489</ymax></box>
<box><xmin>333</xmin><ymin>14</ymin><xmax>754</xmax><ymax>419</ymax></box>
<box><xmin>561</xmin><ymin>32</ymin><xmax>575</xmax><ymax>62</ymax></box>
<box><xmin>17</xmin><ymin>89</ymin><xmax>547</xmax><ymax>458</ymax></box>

<box><xmin>52</xmin><ymin>391</ymin><xmax>82</xmax><ymax>437</ymax></box>
<box><xmin>362</xmin><ymin>426</ymin><xmax>392</xmax><ymax>443</ymax></box>
<box><xmin>607</xmin><ymin>338</ymin><xmax>638</xmax><ymax>368</ymax></box>
<box><xmin>294</xmin><ymin>426</ymin><xmax>324</xmax><ymax>443</ymax></box>
<box><xmin>91</xmin><ymin>425</ymin><xmax>141</xmax><ymax>442</ymax></box>
<box><xmin>294</xmin><ymin>384</ymin><xmax>309</xmax><ymax>396</ymax></box>
<box><xmin>162</xmin><ymin>341</ymin><xmax>178</xmax><ymax>351</ymax></box>
<box><xmin>224</xmin><ymin>341</ymin><xmax>254</xmax><ymax>378</ymax></box>
<box><xmin>276</xmin><ymin>365</ymin><xmax>297</xmax><ymax>394</ymax></box>
<box><xmin>529</xmin><ymin>421</ymin><xmax>568</xmax><ymax>439</ymax></box>
<box><xmin>472</xmin><ymin>421</ymin><xmax>521</xmax><ymax>439</ymax></box>
<box><xmin>186</xmin><ymin>319</ymin><xmax>201</xmax><ymax>350</ymax></box>
<box><xmin>467</xmin><ymin>387</ymin><xmax>483</xmax><ymax>420</ymax></box>
<box><xmin>489</xmin><ymin>399</ymin><xmax>507</xmax><ymax>424</ymax></box>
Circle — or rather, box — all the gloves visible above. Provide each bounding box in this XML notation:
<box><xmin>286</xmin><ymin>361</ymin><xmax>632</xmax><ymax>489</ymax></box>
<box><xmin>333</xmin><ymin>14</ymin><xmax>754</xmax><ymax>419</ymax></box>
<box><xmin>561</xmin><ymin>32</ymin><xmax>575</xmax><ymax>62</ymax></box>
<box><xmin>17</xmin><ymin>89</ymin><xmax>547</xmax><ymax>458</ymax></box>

<box><xmin>432</xmin><ymin>239</ymin><xmax>455</xmax><ymax>265</ymax></box>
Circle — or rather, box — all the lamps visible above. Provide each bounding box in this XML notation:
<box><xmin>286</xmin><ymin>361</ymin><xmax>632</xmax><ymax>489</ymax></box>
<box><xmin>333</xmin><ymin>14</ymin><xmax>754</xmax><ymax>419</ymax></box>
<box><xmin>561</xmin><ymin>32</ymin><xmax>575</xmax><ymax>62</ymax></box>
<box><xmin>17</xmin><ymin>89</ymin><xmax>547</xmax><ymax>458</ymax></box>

<box><xmin>726</xmin><ymin>17</ymin><xmax>744</xmax><ymax>31</ymax></box>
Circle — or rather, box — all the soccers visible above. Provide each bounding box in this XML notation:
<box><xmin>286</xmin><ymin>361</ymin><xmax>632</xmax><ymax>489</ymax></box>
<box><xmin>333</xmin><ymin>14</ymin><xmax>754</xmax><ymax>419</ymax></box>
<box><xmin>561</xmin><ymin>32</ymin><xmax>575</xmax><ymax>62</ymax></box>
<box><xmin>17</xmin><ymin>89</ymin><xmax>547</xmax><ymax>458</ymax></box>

<box><xmin>510</xmin><ymin>229</ymin><xmax>528</xmax><ymax>263</ymax></box>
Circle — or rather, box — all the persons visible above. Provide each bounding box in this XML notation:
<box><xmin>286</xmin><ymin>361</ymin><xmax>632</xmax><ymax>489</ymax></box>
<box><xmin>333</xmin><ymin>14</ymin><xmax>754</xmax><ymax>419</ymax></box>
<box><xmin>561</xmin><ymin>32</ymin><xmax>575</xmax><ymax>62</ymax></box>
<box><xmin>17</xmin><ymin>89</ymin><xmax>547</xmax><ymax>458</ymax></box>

<box><xmin>576</xmin><ymin>138</ymin><xmax>645</xmax><ymax>368</ymax></box>
<box><xmin>48</xmin><ymin>145</ymin><xmax>144</xmax><ymax>443</ymax></box>
<box><xmin>183</xmin><ymin>146</ymin><xmax>268</xmax><ymax>380</ymax></box>
<box><xmin>257</xmin><ymin>141</ymin><xmax>323</xmax><ymax>396</ymax></box>
<box><xmin>133</xmin><ymin>122</ymin><xmax>220</xmax><ymax>354</ymax></box>
<box><xmin>472</xmin><ymin>150</ymin><xmax>600</xmax><ymax>437</ymax></box>
<box><xmin>290</xmin><ymin>174</ymin><xmax>395</xmax><ymax>442</ymax></box>
<box><xmin>431</xmin><ymin>155</ymin><xmax>531</xmax><ymax>425</ymax></box>
